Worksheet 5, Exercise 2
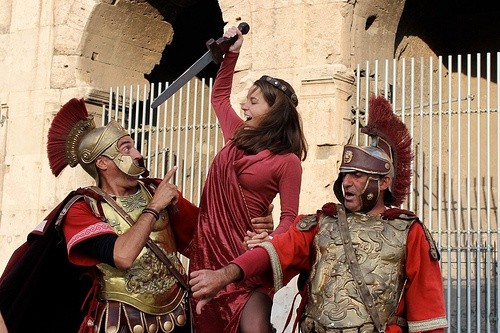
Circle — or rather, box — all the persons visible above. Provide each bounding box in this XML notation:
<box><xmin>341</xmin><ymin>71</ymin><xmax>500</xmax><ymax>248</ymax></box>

<box><xmin>189</xmin><ymin>24</ymin><xmax>308</xmax><ymax>333</ymax></box>
<box><xmin>63</xmin><ymin>121</ymin><xmax>276</xmax><ymax>333</ymax></box>
<box><xmin>188</xmin><ymin>144</ymin><xmax>448</xmax><ymax>333</ymax></box>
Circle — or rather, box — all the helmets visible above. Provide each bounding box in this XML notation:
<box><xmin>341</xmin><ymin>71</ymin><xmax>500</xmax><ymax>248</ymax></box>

<box><xmin>47</xmin><ymin>97</ymin><xmax>150</xmax><ymax>187</ymax></box>
<box><xmin>334</xmin><ymin>94</ymin><xmax>414</xmax><ymax>213</ymax></box>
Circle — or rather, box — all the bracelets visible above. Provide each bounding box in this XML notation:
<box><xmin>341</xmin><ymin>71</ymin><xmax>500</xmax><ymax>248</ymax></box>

<box><xmin>141</xmin><ymin>208</ymin><xmax>160</xmax><ymax>221</ymax></box>
